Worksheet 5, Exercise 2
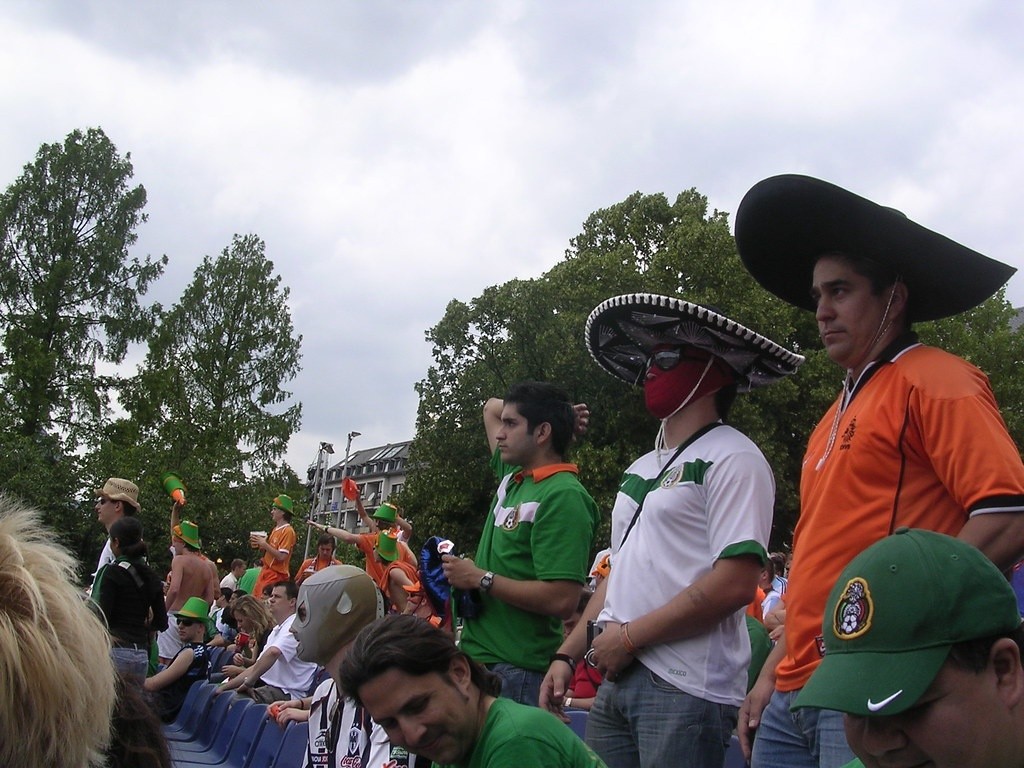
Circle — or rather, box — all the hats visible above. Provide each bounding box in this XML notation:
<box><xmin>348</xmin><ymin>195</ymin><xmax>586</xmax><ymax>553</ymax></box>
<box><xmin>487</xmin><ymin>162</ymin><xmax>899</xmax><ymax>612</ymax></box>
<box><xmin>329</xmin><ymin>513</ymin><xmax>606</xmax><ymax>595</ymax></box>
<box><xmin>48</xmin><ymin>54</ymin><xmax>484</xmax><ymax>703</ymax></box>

<box><xmin>270</xmin><ymin>495</ymin><xmax>295</xmax><ymax>515</ymax></box>
<box><xmin>94</xmin><ymin>478</ymin><xmax>141</xmax><ymax>510</ymax></box>
<box><xmin>373</xmin><ymin>531</ymin><xmax>400</xmax><ymax>562</ymax></box>
<box><xmin>170</xmin><ymin>596</ymin><xmax>211</xmax><ymax>625</ymax></box>
<box><xmin>779</xmin><ymin>528</ymin><xmax>1020</xmax><ymax>716</ymax></box>
<box><xmin>367</xmin><ymin>503</ymin><xmax>396</xmax><ymax>522</ymax></box>
<box><xmin>580</xmin><ymin>293</ymin><xmax>804</xmax><ymax>392</ymax></box>
<box><xmin>171</xmin><ymin>521</ymin><xmax>201</xmax><ymax>549</ymax></box>
<box><xmin>732</xmin><ymin>174</ymin><xmax>1016</xmax><ymax>325</ymax></box>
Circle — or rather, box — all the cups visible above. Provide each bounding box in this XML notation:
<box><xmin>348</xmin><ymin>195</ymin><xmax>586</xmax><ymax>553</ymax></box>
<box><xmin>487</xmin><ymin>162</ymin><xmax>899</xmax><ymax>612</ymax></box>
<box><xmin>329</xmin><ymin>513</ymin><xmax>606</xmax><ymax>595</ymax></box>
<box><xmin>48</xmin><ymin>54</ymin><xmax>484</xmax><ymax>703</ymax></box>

<box><xmin>238</xmin><ymin>632</ymin><xmax>250</xmax><ymax>646</ymax></box>
<box><xmin>586</xmin><ymin>619</ymin><xmax>602</xmax><ymax>667</ymax></box>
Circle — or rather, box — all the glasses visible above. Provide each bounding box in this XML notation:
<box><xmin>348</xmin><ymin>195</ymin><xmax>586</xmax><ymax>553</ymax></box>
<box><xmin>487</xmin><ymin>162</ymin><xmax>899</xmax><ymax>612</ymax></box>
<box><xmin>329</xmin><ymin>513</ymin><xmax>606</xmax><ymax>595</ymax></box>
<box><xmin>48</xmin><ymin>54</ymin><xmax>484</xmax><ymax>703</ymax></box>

<box><xmin>638</xmin><ymin>347</ymin><xmax>716</xmax><ymax>373</ymax></box>
<box><xmin>175</xmin><ymin>616</ymin><xmax>195</xmax><ymax>627</ymax></box>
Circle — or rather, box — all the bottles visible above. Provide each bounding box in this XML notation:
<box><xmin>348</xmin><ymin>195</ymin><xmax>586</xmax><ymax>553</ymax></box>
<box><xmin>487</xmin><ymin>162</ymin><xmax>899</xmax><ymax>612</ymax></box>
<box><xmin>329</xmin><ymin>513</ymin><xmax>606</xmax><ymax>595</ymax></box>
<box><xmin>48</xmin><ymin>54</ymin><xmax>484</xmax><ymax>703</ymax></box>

<box><xmin>272</xmin><ymin>705</ymin><xmax>280</xmax><ymax>722</ymax></box>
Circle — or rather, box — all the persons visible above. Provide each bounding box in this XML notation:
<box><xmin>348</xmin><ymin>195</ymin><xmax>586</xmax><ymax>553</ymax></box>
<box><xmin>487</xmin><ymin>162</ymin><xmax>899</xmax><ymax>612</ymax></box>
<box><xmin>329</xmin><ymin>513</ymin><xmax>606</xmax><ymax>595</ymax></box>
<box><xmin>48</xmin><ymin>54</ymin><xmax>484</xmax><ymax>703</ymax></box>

<box><xmin>735</xmin><ymin>171</ymin><xmax>1024</xmax><ymax>768</ymax></box>
<box><xmin>1</xmin><ymin>489</ymin><xmax>117</xmax><ymax>768</ymax></box>
<box><xmin>538</xmin><ymin>287</ymin><xmax>779</xmax><ymax>768</ymax></box>
<box><xmin>789</xmin><ymin>521</ymin><xmax>1024</xmax><ymax>768</ymax></box>
<box><xmin>440</xmin><ymin>377</ymin><xmax>604</xmax><ymax>716</ymax></box>
<box><xmin>77</xmin><ymin>454</ymin><xmax>798</xmax><ymax>768</ymax></box>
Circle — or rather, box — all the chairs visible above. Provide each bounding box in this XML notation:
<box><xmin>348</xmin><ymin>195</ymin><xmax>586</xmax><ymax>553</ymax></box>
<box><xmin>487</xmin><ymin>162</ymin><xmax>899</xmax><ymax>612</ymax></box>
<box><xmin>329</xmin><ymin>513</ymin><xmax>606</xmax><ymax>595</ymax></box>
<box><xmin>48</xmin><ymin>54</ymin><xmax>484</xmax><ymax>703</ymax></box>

<box><xmin>153</xmin><ymin>642</ymin><xmax>312</xmax><ymax>768</ymax></box>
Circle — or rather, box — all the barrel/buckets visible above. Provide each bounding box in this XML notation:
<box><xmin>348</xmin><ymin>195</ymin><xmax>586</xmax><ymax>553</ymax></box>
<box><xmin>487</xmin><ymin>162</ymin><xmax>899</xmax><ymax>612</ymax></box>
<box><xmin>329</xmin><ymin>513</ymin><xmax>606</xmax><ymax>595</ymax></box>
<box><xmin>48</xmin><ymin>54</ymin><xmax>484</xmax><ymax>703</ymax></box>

<box><xmin>249</xmin><ymin>531</ymin><xmax>268</xmax><ymax>549</ymax></box>
<box><xmin>302</xmin><ymin>572</ymin><xmax>315</xmax><ymax>579</ymax></box>
